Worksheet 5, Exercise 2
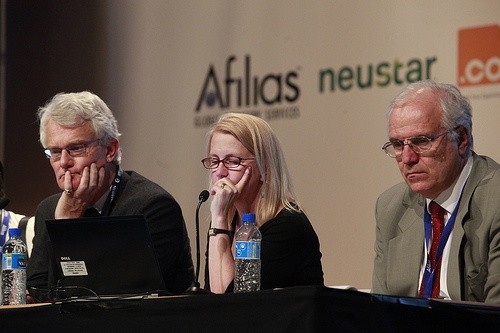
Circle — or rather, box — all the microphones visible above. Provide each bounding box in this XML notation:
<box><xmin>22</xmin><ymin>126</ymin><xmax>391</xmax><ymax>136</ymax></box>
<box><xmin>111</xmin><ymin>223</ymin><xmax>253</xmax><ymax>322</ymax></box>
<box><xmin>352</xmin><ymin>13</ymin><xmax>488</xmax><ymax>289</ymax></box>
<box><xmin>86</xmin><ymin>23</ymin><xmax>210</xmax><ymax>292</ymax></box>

<box><xmin>185</xmin><ymin>190</ymin><xmax>210</xmax><ymax>295</ymax></box>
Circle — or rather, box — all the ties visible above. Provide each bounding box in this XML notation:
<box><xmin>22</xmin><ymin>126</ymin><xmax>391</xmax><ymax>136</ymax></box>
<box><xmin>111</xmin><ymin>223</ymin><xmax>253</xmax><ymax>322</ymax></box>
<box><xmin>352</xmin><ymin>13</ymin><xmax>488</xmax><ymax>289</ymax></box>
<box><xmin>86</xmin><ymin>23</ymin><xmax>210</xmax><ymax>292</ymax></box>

<box><xmin>417</xmin><ymin>202</ymin><xmax>446</xmax><ymax>297</ymax></box>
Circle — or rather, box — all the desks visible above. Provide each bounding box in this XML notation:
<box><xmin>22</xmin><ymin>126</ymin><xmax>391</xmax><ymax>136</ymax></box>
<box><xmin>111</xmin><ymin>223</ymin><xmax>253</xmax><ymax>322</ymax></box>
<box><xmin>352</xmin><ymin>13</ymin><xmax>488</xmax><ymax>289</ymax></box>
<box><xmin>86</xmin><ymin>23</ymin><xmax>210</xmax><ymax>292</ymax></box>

<box><xmin>0</xmin><ymin>286</ymin><xmax>500</xmax><ymax>333</ymax></box>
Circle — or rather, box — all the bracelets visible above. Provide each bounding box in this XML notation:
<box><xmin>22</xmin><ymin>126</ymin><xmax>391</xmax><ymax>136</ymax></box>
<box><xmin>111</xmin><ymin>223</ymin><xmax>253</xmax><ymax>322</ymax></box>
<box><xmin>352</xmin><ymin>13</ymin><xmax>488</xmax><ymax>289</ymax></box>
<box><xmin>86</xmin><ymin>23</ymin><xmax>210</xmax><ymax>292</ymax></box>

<box><xmin>208</xmin><ymin>227</ymin><xmax>230</xmax><ymax>237</ymax></box>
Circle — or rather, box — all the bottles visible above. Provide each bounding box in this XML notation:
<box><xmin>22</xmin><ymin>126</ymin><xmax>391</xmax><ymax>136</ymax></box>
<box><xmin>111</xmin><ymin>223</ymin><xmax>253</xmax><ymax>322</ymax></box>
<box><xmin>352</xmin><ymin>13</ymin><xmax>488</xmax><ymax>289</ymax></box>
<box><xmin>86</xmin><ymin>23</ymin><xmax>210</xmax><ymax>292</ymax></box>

<box><xmin>0</xmin><ymin>228</ymin><xmax>29</xmax><ymax>306</ymax></box>
<box><xmin>234</xmin><ymin>213</ymin><xmax>262</xmax><ymax>292</ymax></box>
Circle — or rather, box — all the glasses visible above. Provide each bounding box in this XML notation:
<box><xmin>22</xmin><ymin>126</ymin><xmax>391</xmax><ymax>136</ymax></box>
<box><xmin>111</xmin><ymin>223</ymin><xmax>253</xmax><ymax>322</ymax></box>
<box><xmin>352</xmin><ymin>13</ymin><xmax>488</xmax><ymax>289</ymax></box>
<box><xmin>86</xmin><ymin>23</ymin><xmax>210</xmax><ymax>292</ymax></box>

<box><xmin>381</xmin><ymin>126</ymin><xmax>458</xmax><ymax>158</ymax></box>
<box><xmin>40</xmin><ymin>137</ymin><xmax>102</xmax><ymax>159</ymax></box>
<box><xmin>201</xmin><ymin>155</ymin><xmax>256</xmax><ymax>170</ymax></box>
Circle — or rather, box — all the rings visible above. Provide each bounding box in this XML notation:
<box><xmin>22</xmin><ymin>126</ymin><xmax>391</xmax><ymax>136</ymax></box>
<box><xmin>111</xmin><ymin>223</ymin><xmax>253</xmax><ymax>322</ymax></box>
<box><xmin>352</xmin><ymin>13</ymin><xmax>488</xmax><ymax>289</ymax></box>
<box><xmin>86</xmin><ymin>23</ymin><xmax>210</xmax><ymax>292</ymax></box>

<box><xmin>64</xmin><ymin>188</ymin><xmax>73</xmax><ymax>195</ymax></box>
<box><xmin>220</xmin><ymin>182</ymin><xmax>227</xmax><ymax>189</ymax></box>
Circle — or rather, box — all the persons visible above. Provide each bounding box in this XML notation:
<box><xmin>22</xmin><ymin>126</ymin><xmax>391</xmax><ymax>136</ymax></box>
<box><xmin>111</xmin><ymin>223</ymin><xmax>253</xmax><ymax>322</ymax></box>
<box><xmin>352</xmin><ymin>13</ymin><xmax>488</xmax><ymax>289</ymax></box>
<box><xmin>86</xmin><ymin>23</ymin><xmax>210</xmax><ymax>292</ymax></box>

<box><xmin>199</xmin><ymin>112</ymin><xmax>325</xmax><ymax>296</ymax></box>
<box><xmin>369</xmin><ymin>81</ymin><xmax>500</xmax><ymax>308</ymax></box>
<box><xmin>22</xmin><ymin>90</ymin><xmax>196</xmax><ymax>304</ymax></box>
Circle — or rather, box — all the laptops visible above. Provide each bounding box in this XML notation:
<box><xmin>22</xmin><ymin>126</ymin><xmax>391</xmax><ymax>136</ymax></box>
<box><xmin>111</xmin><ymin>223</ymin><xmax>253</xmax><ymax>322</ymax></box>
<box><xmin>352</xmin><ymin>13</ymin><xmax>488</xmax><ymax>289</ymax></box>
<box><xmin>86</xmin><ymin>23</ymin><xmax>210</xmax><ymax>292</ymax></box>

<box><xmin>44</xmin><ymin>215</ymin><xmax>176</xmax><ymax>297</ymax></box>
